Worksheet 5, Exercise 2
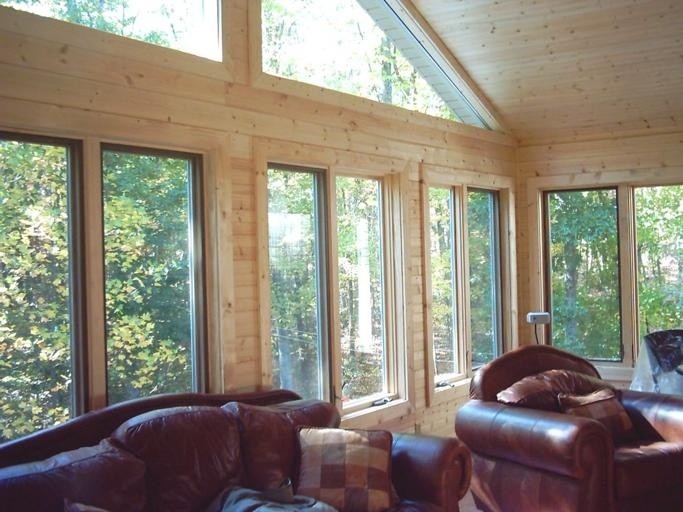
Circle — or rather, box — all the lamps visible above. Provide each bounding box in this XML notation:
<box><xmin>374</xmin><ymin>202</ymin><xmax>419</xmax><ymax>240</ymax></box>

<box><xmin>527</xmin><ymin>311</ymin><xmax>552</xmax><ymax>344</ymax></box>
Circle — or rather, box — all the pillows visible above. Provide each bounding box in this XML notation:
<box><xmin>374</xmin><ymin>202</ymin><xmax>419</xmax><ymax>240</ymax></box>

<box><xmin>292</xmin><ymin>422</ymin><xmax>402</xmax><ymax>512</ymax></box>
<box><xmin>556</xmin><ymin>384</ymin><xmax>638</xmax><ymax>447</ymax></box>
<box><xmin>496</xmin><ymin>368</ymin><xmax>623</xmax><ymax>416</ymax></box>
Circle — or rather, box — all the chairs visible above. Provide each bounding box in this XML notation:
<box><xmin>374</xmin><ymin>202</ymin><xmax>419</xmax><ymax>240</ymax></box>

<box><xmin>630</xmin><ymin>327</ymin><xmax>682</xmax><ymax>397</ymax></box>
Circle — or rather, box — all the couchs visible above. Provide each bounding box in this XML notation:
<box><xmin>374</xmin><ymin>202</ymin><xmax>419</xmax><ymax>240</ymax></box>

<box><xmin>0</xmin><ymin>389</ymin><xmax>472</xmax><ymax>512</ymax></box>
<box><xmin>451</xmin><ymin>342</ymin><xmax>682</xmax><ymax>512</ymax></box>
